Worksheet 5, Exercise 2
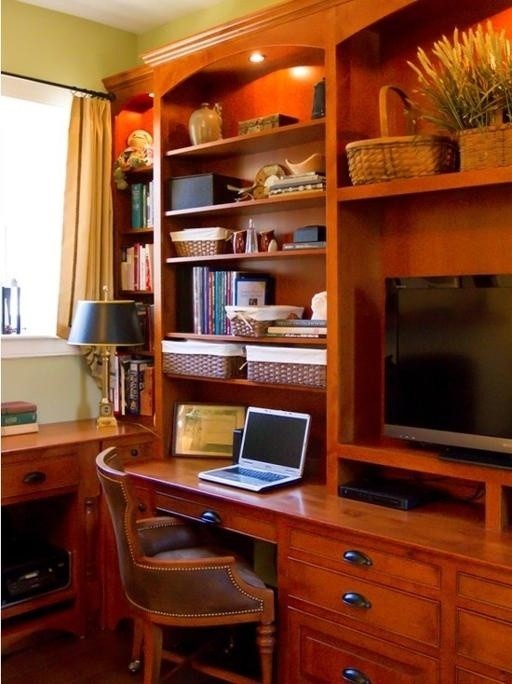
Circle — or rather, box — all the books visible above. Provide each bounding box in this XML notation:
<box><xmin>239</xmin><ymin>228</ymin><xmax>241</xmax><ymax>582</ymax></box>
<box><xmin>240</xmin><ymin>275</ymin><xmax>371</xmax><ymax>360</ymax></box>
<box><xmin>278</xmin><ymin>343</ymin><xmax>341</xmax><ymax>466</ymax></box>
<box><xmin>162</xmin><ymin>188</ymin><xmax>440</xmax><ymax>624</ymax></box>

<box><xmin>131</xmin><ymin>179</ymin><xmax>154</xmax><ymax>228</ymax></box>
<box><xmin>1</xmin><ymin>401</ymin><xmax>40</xmax><ymax>437</ymax></box>
<box><xmin>282</xmin><ymin>241</ymin><xmax>325</xmax><ymax>249</ymax></box>
<box><xmin>269</xmin><ymin>171</ymin><xmax>326</xmax><ymax>198</ymax></box>
<box><xmin>108</xmin><ymin>353</ymin><xmax>156</xmax><ymax>418</ymax></box>
<box><xmin>136</xmin><ymin>301</ymin><xmax>155</xmax><ymax>351</ymax></box>
<box><xmin>117</xmin><ymin>241</ymin><xmax>153</xmax><ymax>291</ymax></box>
<box><xmin>193</xmin><ymin>264</ymin><xmax>326</xmax><ymax>340</ymax></box>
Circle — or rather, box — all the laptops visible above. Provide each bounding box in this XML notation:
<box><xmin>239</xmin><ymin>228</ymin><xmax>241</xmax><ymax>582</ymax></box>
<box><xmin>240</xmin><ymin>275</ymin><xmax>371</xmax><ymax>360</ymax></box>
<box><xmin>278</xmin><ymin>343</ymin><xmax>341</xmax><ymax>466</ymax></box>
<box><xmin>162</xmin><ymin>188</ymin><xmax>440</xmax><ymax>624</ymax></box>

<box><xmin>201</xmin><ymin>411</ymin><xmax>312</xmax><ymax>492</ymax></box>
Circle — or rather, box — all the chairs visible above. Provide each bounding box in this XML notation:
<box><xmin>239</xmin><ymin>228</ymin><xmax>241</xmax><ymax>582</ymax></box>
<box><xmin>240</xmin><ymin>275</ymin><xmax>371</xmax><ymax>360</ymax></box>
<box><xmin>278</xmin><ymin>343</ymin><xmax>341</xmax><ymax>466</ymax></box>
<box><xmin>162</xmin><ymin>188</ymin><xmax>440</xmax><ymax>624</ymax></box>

<box><xmin>96</xmin><ymin>445</ymin><xmax>275</xmax><ymax>683</ymax></box>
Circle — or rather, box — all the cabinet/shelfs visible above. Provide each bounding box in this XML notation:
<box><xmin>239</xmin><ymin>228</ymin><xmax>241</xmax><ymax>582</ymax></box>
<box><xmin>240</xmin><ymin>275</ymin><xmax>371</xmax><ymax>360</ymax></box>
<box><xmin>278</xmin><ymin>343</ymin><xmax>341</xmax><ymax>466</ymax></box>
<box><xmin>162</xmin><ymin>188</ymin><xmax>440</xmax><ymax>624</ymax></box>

<box><xmin>1</xmin><ymin>413</ymin><xmax>158</xmax><ymax>655</ymax></box>
<box><xmin>100</xmin><ymin>0</ymin><xmax>511</xmax><ymax>684</ymax></box>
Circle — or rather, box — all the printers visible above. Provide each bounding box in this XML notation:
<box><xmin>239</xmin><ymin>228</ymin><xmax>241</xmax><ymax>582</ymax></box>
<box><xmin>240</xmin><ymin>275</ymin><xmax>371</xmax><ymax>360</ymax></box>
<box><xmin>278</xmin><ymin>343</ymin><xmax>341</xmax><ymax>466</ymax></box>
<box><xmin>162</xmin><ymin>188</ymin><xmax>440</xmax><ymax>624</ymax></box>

<box><xmin>1</xmin><ymin>539</ymin><xmax>73</xmax><ymax>610</ymax></box>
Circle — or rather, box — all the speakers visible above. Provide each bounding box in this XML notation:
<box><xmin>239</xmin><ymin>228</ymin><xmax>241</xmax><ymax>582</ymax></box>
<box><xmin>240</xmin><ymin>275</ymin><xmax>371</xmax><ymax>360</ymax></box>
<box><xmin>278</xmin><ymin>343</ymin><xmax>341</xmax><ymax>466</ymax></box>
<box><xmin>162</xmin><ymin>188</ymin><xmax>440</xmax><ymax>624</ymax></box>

<box><xmin>233</xmin><ymin>430</ymin><xmax>243</xmax><ymax>466</ymax></box>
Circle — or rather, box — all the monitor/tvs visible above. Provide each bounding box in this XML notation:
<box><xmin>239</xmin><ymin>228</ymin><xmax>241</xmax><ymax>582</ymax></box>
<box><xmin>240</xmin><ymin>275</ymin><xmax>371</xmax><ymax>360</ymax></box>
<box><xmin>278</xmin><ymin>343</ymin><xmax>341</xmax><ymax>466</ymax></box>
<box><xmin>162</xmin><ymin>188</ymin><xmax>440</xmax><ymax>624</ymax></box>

<box><xmin>384</xmin><ymin>273</ymin><xmax>512</xmax><ymax>470</ymax></box>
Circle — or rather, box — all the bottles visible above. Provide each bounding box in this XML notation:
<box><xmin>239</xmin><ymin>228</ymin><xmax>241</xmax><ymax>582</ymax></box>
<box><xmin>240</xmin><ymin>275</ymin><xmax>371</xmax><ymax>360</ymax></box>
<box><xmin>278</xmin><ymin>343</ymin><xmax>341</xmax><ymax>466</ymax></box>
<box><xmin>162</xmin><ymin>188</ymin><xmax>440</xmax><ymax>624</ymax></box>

<box><xmin>267</xmin><ymin>240</ymin><xmax>278</xmax><ymax>252</ymax></box>
<box><xmin>311</xmin><ymin>77</ymin><xmax>326</xmax><ymax>119</ymax></box>
<box><xmin>189</xmin><ymin>103</ymin><xmax>220</xmax><ymax>146</ymax></box>
<box><xmin>245</xmin><ymin>217</ymin><xmax>259</xmax><ymax>254</ymax></box>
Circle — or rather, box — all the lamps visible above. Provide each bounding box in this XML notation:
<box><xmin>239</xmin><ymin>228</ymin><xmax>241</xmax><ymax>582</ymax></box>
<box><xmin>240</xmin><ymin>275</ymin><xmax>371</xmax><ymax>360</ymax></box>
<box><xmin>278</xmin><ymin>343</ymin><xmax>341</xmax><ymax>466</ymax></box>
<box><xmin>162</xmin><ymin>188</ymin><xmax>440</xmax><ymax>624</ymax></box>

<box><xmin>67</xmin><ymin>300</ymin><xmax>146</xmax><ymax>427</ymax></box>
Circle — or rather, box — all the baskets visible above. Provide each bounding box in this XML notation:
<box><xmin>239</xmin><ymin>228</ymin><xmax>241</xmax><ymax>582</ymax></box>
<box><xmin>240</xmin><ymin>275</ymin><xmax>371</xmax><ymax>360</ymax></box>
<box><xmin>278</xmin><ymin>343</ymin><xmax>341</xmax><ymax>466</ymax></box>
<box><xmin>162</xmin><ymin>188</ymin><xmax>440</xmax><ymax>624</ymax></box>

<box><xmin>170</xmin><ymin>227</ymin><xmax>229</xmax><ymax>256</ymax></box>
<box><xmin>225</xmin><ymin>305</ymin><xmax>303</xmax><ymax>338</ymax></box>
<box><xmin>345</xmin><ymin>123</ymin><xmax>512</xmax><ymax>186</ymax></box>
<box><xmin>162</xmin><ymin>340</ymin><xmax>327</xmax><ymax>386</ymax></box>
